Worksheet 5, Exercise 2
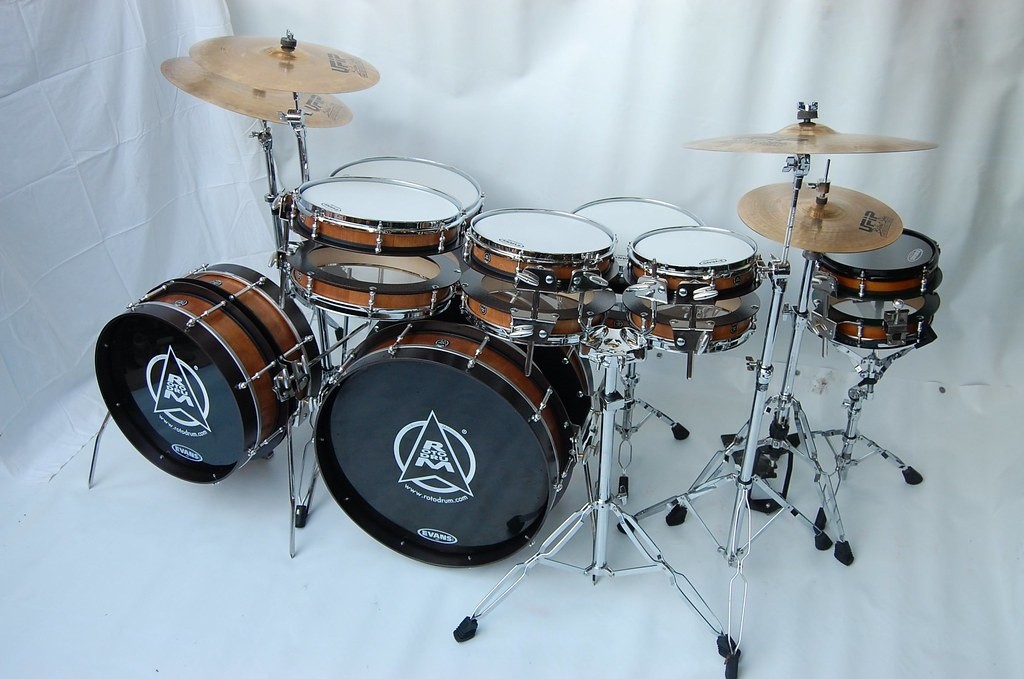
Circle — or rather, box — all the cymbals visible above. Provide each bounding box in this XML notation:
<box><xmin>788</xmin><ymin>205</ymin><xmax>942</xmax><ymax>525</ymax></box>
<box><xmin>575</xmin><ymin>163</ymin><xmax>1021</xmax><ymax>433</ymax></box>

<box><xmin>189</xmin><ymin>36</ymin><xmax>381</xmax><ymax>94</ymax></box>
<box><xmin>160</xmin><ymin>55</ymin><xmax>353</xmax><ymax>128</ymax></box>
<box><xmin>682</xmin><ymin>123</ymin><xmax>939</xmax><ymax>154</ymax></box>
<box><xmin>737</xmin><ymin>181</ymin><xmax>904</xmax><ymax>254</ymax></box>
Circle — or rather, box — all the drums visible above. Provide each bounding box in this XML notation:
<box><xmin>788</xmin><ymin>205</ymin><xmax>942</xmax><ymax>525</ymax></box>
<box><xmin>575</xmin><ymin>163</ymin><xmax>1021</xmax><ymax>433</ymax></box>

<box><xmin>809</xmin><ymin>229</ymin><xmax>942</xmax><ymax>353</ymax></box>
<box><xmin>93</xmin><ymin>260</ymin><xmax>322</xmax><ymax>485</ymax></box>
<box><xmin>312</xmin><ymin>297</ymin><xmax>597</xmax><ymax>575</ymax></box>
<box><xmin>571</xmin><ymin>197</ymin><xmax>707</xmax><ymax>330</ymax></box>
<box><xmin>626</xmin><ymin>225</ymin><xmax>762</xmax><ymax>354</ymax></box>
<box><xmin>456</xmin><ymin>206</ymin><xmax>622</xmax><ymax>349</ymax></box>
<box><xmin>330</xmin><ymin>155</ymin><xmax>485</xmax><ymax>298</ymax></box>
<box><xmin>287</xmin><ymin>175</ymin><xmax>466</xmax><ymax>321</ymax></box>
